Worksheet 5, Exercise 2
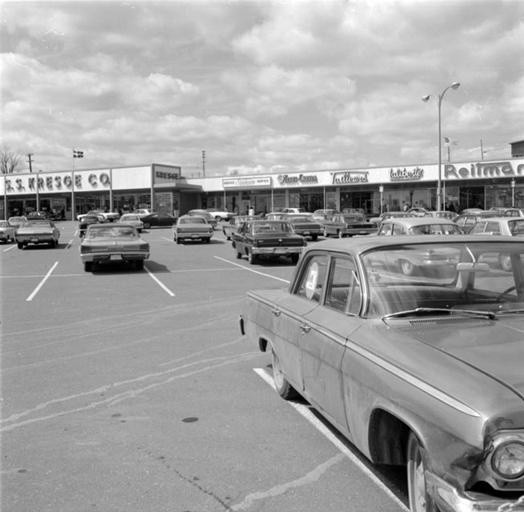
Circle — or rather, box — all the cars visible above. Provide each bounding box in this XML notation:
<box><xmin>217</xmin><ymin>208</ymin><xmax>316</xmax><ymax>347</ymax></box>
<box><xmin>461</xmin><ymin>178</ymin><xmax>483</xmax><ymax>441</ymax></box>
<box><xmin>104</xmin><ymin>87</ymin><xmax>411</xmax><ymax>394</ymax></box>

<box><xmin>238</xmin><ymin>234</ymin><xmax>524</xmax><ymax>511</ymax></box>
<box><xmin>0</xmin><ymin>206</ymin><xmax>66</xmax><ymax>249</ymax></box>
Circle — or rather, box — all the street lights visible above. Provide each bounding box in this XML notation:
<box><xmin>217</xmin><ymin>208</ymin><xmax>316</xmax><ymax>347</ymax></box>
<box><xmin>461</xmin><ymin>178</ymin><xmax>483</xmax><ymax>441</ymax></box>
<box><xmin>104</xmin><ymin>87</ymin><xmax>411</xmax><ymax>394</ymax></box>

<box><xmin>35</xmin><ymin>169</ymin><xmax>42</xmax><ymax>213</ymax></box>
<box><xmin>71</xmin><ymin>149</ymin><xmax>85</xmax><ymax>222</ymax></box>
<box><xmin>421</xmin><ymin>82</ymin><xmax>462</xmax><ymax>212</ymax></box>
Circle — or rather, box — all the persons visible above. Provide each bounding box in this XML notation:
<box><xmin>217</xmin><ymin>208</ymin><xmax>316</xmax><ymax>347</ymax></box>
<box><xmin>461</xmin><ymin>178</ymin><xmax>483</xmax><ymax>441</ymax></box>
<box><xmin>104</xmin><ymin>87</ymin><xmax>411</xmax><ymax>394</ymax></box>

<box><xmin>379</xmin><ymin>195</ymin><xmax>483</xmax><ymax>215</ymax></box>
<box><xmin>248</xmin><ymin>204</ymin><xmax>254</xmax><ymax>216</ymax></box>
